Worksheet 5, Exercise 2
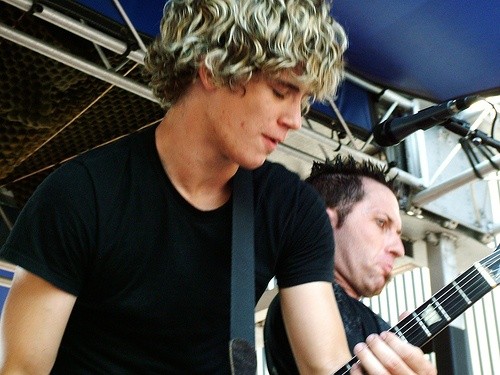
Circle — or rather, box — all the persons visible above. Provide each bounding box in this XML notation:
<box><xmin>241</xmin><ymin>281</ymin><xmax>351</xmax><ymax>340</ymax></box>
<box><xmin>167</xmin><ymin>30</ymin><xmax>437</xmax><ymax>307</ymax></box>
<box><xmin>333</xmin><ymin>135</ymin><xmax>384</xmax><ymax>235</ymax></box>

<box><xmin>263</xmin><ymin>151</ymin><xmax>407</xmax><ymax>375</ymax></box>
<box><xmin>0</xmin><ymin>0</ymin><xmax>439</xmax><ymax>374</ymax></box>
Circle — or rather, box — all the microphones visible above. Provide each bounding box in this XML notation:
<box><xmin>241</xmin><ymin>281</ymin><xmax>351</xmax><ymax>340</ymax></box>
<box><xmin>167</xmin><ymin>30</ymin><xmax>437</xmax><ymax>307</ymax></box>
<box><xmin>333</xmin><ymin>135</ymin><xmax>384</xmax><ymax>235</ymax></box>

<box><xmin>374</xmin><ymin>96</ymin><xmax>480</xmax><ymax>147</ymax></box>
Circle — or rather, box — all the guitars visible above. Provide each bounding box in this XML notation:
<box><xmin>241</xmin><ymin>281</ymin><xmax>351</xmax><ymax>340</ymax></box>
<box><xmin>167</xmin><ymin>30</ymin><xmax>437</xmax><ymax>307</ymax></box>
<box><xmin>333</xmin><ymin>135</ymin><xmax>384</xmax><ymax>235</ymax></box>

<box><xmin>330</xmin><ymin>246</ymin><xmax>500</xmax><ymax>375</ymax></box>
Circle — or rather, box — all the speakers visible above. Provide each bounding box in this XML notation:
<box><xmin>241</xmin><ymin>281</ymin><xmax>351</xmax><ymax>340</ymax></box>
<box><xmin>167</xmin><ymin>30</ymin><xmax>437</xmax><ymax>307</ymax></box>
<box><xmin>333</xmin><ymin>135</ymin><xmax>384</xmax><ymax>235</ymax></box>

<box><xmin>434</xmin><ymin>326</ymin><xmax>473</xmax><ymax>375</ymax></box>
